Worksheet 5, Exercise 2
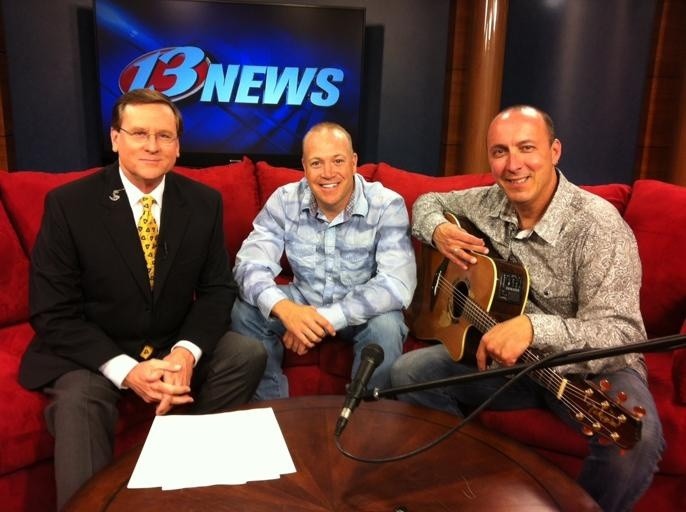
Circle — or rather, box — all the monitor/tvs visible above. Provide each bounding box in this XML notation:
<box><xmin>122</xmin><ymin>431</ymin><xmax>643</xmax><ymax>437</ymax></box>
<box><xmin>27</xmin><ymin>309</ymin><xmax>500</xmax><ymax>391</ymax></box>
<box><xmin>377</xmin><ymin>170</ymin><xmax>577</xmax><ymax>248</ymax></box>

<box><xmin>77</xmin><ymin>0</ymin><xmax>370</xmax><ymax>167</ymax></box>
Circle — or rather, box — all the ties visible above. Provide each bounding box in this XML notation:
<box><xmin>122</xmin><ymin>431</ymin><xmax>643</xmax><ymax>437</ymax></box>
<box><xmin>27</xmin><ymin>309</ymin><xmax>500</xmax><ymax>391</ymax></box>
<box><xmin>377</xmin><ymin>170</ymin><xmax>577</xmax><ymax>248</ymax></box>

<box><xmin>136</xmin><ymin>197</ymin><xmax>159</xmax><ymax>290</ymax></box>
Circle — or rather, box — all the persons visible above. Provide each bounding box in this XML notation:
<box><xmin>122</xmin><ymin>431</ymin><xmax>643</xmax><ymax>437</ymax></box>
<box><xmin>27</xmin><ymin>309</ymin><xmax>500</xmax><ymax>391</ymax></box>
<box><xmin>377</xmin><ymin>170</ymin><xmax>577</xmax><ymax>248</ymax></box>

<box><xmin>14</xmin><ymin>86</ymin><xmax>268</xmax><ymax>512</ymax></box>
<box><xmin>228</xmin><ymin>122</ymin><xmax>419</xmax><ymax>405</ymax></box>
<box><xmin>391</xmin><ymin>104</ymin><xmax>668</xmax><ymax>512</ymax></box>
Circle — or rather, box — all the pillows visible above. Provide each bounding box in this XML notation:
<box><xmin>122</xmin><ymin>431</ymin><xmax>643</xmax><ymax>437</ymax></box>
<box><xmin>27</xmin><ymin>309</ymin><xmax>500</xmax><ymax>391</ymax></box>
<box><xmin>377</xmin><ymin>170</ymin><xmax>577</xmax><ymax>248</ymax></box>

<box><xmin>0</xmin><ymin>156</ymin><xmax>686</xmax><ymax>326</ymax></box>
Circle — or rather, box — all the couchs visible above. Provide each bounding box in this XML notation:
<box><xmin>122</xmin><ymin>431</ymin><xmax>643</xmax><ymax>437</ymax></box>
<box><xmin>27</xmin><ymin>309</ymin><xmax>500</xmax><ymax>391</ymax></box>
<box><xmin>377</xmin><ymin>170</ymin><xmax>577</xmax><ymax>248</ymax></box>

<box><xmin>0</xmin><ymin>324</ymin><xmax>686</xmax><ymax>512</ymax></box>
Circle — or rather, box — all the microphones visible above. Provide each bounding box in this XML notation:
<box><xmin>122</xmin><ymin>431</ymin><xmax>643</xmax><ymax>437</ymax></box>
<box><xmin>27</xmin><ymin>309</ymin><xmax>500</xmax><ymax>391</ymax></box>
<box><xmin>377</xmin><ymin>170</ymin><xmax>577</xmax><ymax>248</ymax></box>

<box><xmin>334</xmin><ymin>343</ymin><xmax>384</xmax><ymax>436</ymax></box>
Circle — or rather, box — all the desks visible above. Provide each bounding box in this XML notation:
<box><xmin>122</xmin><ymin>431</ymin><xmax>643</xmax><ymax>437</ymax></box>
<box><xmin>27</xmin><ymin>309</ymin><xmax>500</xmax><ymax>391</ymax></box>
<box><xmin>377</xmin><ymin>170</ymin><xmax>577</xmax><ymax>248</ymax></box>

<box><xmin>60</xmin><ymin>394</ymin><xmax>601</xmax><ymax>511</ymax></box>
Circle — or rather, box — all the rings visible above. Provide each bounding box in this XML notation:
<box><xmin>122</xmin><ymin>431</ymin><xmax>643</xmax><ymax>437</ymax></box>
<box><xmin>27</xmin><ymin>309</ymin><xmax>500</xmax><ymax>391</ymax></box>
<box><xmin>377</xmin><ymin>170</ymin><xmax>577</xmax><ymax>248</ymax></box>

<box><xmin>453</xmin><ymin>247</ymin><xmax>462</xmax><ymax>255</ymax></box>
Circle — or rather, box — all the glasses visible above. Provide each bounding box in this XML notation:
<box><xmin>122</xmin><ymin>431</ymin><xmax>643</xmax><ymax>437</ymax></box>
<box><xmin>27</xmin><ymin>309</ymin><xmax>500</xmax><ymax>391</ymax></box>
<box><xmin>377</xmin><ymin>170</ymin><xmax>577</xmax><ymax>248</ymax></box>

<box><xmin>120</xmin><ymin>125</ymin><xmax>179</xmax><ymax>143</ymax></box>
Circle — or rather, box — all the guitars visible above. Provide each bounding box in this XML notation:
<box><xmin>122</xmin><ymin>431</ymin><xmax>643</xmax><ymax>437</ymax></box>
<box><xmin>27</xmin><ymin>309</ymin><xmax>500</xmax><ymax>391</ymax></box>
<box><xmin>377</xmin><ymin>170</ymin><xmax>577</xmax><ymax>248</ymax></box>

<box><xmin>409</xmin><ymin>211</ymin><xmax>646</xmax><ymax>458</ymax></box>
